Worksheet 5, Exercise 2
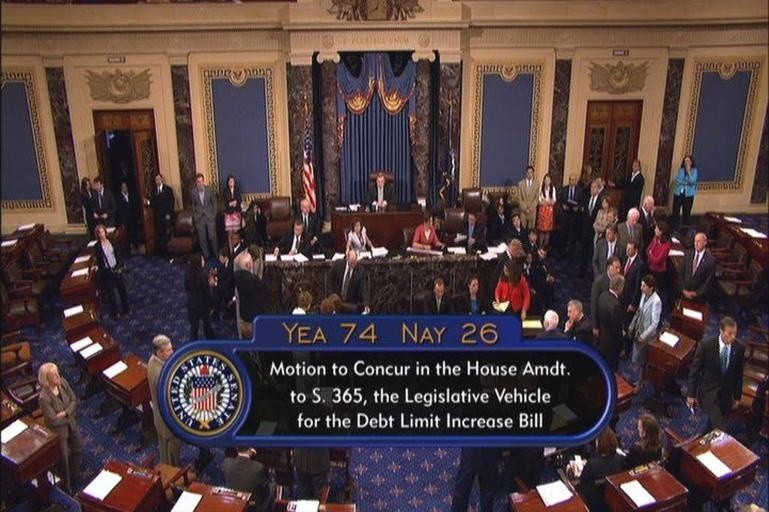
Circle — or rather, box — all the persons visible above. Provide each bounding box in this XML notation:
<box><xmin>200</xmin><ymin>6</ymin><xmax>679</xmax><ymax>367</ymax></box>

<box><xmin>35</xmin><ymin>152</ymin><xmax>768</xmax><ymax>512</ymax></box>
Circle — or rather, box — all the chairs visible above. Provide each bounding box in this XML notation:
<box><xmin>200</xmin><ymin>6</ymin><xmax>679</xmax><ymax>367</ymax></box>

<box><xmin>0</xmin><ymin>172</ymin><xmax>768</xmax><ymax>512</ymax></box>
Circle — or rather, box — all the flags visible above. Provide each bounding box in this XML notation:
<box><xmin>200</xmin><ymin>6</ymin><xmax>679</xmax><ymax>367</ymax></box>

<box><xmin>300</xmin><ymin>101</ymin><xmax>317</xmax><ymax>215</ymax></box>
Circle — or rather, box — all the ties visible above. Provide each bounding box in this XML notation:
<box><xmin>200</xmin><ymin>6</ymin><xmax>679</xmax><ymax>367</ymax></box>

<box><xmin>570</xmin><ymin>187</ymin><xmax>572</xmax><ymax>201</ymax></box>
<box><xmin>625</xmin><ymin>259</ymin><xmax>631</xmax><ymax>274</ymax></box>
<box><xmin>631</xmin><ymin>225</ymin><xmax>635</xmax><ymax>238</ymax></box>
<box><xmin>609</xmin><ymin>243</ymin><xmax>612</xmax><ymax>255</ymax></box>
<box><xmin>304</xmin><ymin>214</ymin><xmax>307</xmax><ymax>231</ymax></box>
<box><xmin>343</xmin><ymin>268</ymin><xmax>351</xmax><ymax>298</ymax></box>
<box><xmin>721</xmin><ymin>345</ymin><xmax>728</xmax><ymax>375</ymax></box>
<box><xmin>590</xmin><ymin>196</ymin><xmax>594</xmax><ymax>214</ymax></box>
<box><xmin>296</xmin><ymin>235</ymin><xmax>298</xmax><ymax>249</ymax></box>
<box><xmin>694</xmin><ymin>253</ymin><xmax>699</xmax><ymax>272</ymax></box>
